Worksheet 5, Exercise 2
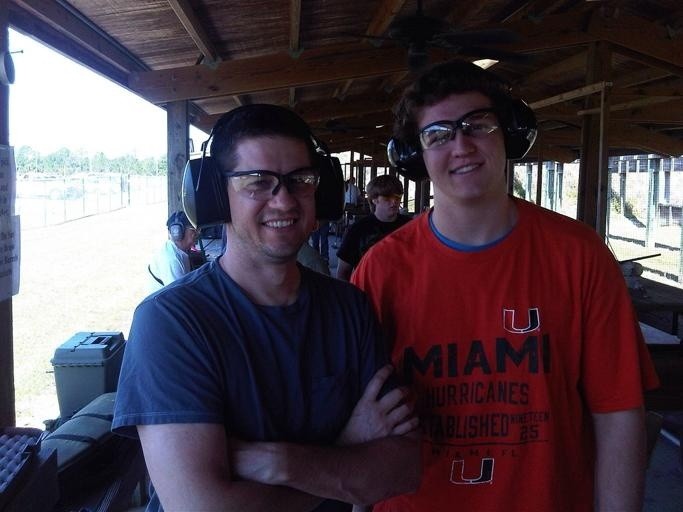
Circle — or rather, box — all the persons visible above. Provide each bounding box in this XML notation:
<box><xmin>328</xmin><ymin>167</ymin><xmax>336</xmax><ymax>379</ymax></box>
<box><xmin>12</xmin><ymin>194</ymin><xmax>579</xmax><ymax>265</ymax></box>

<box><xmin>351</xmin><ymin>60</ymin><xmax>661</xmax><ymax>511</ymax></box>
<box><xmin>110</xmin><ymin>102</ymin><xmax>424</xmax><ymax>512</ymax></box>
<box><xmin>143</xmin><ymin>211</ymin><xmax>200</xmax><ymax>298</ymax></box>
<box><xmin>335</xmin><ymin>174</ymin><xmax>414</xmax><ymax>282</ymax></box>
<box><xmin>311</xmin><ymin>222</ymin><xmax>329</xmax><ymax>263</ymax></box>
<box><xmin>344</xmin><ymin>176</ymin><xmax>365</xmax><ymax>218</ymax></box>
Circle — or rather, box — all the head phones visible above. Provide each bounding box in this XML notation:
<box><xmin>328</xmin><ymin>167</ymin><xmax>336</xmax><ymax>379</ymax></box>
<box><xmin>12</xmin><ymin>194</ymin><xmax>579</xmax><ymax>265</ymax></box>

<box><xmin>181</xmin><ymin>102</ymin><xmax>345</xmax><ymax>229</ymax></box>
<box><xmin>169</xmin><ymin>209</ymin><xmax>184</xmax><ymax>240</ymax></box>
<box><xmin>386</xmin><ymin>68</ymin><xmax>539</xmax><ymax>183</ymax></box>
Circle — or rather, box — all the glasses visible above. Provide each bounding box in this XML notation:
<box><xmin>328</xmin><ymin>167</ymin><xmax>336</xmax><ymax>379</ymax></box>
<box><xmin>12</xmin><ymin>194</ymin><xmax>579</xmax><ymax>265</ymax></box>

<box><xmin>419</xmin><ymin>108</ymin><xmax>498</xmax><ymax>150</ymax></box>
<box><xmin>222</xmin><ymin>166</ymin><xmax>320</xmax><ymax>198</ymax></box>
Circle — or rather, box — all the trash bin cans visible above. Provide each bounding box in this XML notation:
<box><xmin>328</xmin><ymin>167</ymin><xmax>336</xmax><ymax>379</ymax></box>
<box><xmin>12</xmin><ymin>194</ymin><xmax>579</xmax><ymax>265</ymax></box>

<box><xmin>51</xmin><ymin>331</ymin><xmax>126</xmax><ymax>419</ymax></box>
